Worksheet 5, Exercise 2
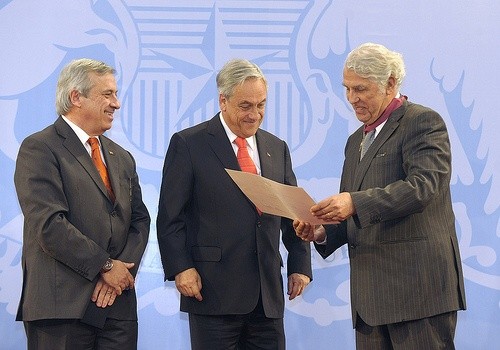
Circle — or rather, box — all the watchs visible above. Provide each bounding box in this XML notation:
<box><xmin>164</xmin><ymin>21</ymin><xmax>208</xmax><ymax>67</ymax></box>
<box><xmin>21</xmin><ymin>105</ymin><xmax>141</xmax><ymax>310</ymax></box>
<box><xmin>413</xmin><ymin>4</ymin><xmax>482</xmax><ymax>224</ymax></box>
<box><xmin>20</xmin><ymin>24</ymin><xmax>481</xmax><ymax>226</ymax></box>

<box><xmin>101</xmin><ymin>258</ymin><xmax>114</xmax><ymax>273</ymax></box>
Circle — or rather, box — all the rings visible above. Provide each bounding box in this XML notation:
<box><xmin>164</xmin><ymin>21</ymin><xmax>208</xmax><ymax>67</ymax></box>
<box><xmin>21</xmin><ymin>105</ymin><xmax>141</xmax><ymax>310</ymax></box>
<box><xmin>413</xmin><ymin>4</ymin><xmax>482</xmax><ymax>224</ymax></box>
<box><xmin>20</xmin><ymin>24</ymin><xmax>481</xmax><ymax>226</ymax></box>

<box><xmin>328</xmin><ymin>213</ymin><xmax>331</xmax><ymax>219</ymax></box>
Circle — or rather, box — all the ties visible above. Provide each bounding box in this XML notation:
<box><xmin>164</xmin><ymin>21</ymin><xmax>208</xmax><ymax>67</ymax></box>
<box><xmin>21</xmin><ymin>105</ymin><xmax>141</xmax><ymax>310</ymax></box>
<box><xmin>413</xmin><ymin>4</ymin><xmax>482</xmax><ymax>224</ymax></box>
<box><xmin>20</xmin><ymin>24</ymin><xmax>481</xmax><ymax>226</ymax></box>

<box><xmin>234</xmin><ymin>137</ymin><xmax>263</xmax><ymax>216</ymax></box>
<box><xmin>86</xmin><ymin>137</ymin><xmax>115</xmax><ymax>202</ymax></box>
<box><xmin>359</xmin><ymin>128</ymin><xmax>376</xmax><ymax>161</ymax></box>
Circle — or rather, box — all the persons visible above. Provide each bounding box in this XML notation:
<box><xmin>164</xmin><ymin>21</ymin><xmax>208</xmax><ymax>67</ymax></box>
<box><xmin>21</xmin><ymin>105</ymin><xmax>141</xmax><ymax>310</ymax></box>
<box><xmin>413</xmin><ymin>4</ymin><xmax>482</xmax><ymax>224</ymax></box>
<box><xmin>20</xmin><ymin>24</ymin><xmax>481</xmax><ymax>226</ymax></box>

<box><xmin>14</xmin><ymin>59</ymin><xmax>151</xmax><ymax>350</ymax></box>
<box><xmin>292</xmin><ymin>41</ymin><xmax>466</xmax><ymax>350</ymax></box>
<box><xmin>156</xmin><ymin>59</ymin><xmax>314</xmax><ymax>350</ymax></box>
